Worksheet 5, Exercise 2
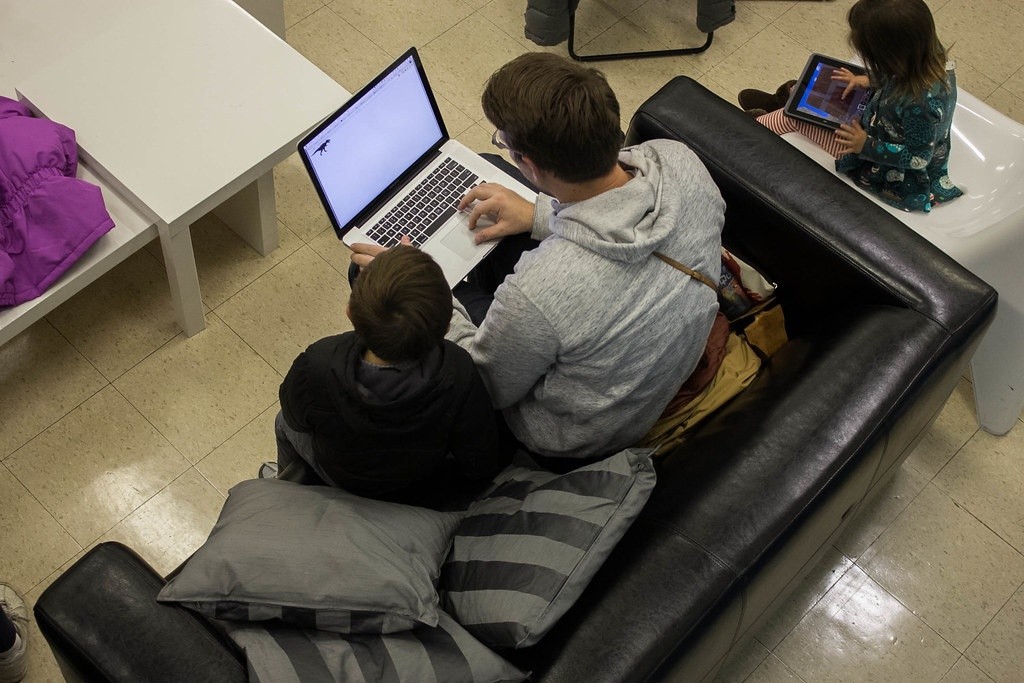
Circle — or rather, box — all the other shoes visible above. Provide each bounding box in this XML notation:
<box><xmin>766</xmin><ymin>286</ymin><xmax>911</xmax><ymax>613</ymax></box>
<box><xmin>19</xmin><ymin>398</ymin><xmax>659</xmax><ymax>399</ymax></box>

<box><xmin>258</xmin><ymin>460</ymin><xmax>278</xmax><ymax>478</ymax></box>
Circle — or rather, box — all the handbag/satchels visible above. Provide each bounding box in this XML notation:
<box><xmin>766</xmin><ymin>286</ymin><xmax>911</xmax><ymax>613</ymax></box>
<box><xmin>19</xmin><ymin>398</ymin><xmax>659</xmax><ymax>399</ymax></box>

<box><xmin>719</xmin><ymin>244</ymin><xmax>787</xmax><ymax>358</ymax></box>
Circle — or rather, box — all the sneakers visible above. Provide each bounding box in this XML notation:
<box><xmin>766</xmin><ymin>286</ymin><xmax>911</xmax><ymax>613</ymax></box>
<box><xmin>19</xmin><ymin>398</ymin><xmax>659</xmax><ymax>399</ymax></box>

<box><xmin>0</xmin><ymin>581</ymin><xmax>30</xmax><ymax>683</ymax></box>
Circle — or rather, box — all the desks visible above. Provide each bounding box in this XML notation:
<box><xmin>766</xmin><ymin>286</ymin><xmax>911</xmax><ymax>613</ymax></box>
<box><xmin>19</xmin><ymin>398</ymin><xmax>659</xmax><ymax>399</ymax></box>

<box><xmin>1</xmin><ymin>1</ymin><xmax>355</xmax><ymax>341</ymax></box>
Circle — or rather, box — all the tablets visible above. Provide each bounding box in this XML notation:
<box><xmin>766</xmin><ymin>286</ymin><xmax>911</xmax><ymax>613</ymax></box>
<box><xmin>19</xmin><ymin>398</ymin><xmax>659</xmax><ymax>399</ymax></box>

<box><xmin>784</xmin><ymin>53</ymin><xmax>876</xmax><ymax>132</ymax></box>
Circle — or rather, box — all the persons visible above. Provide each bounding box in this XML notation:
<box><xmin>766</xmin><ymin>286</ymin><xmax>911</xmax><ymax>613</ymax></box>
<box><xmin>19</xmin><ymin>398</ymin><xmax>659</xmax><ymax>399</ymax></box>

<box><xmin>258</xmin><ymin>244</ymin><xmax>500</xmax><ymax>513</ymax></box>
<box><xmin>346</xmin><ymin>52</ymin><xmax>726</xmax><ymax>475</ymax></box>
<box><xmin>738</xmin><ymin>0</ymin><xmax>963</xmax><ymax>214</ymax></box>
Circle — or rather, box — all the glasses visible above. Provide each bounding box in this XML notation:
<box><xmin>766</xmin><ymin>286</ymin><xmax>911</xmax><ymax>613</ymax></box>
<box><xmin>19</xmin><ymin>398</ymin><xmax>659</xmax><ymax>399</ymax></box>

<box><xmin>491</xmin><ymin>127</ymin><xmax>525</xmax><ymax>156</ymax></box>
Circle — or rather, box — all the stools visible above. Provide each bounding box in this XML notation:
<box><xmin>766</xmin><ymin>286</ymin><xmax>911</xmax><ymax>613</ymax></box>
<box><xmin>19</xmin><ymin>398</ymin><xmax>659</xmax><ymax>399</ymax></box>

<box><xmin>779</xmin><ymin>86</ymin><xmax>1024</xmax><ymax>438</ymax></box>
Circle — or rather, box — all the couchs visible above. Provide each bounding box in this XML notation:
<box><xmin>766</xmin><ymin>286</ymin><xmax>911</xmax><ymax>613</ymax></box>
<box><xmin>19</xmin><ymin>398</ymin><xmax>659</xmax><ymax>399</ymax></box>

<box><xmin>32</xmin><ymin>75</ymin><xmax>998</xmax><ymax>683</ymax></box>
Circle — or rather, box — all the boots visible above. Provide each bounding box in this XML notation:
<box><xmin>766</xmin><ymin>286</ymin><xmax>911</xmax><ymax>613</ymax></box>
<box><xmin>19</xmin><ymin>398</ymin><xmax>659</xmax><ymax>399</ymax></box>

<box><xmin>744</xmin><ymin>110</ymin><xmax>766</xmax><ymax>119</ymax></box>
<box><xmin>739</xmin><ymin>80</ymin><xmax>798</xmax><ymax>113</ymax></box>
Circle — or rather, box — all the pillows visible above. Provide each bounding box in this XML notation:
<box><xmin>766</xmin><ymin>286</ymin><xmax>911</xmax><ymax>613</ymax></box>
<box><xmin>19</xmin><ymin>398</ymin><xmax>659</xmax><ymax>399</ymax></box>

<box><xmin>154</xmin><ymin>479</ymin><xmax>464</xmax><ymax>636</ymax></box>
<box><xmin>440</xmin><ymin>448</ymin><xmax>659</xmax><ymax>649</ymax></box>
<box><xmin>205</xmin><ymin>605</ymin><xmax>537</xmax><ymax>683</ymax></box>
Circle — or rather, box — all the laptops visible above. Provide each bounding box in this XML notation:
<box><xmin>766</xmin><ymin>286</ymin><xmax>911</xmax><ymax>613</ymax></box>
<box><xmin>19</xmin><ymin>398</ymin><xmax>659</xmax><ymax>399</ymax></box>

<box><xmin>296</xmin><ymin>46</ymin><xmax>537</xmax><ymax>290</ymax></box>
